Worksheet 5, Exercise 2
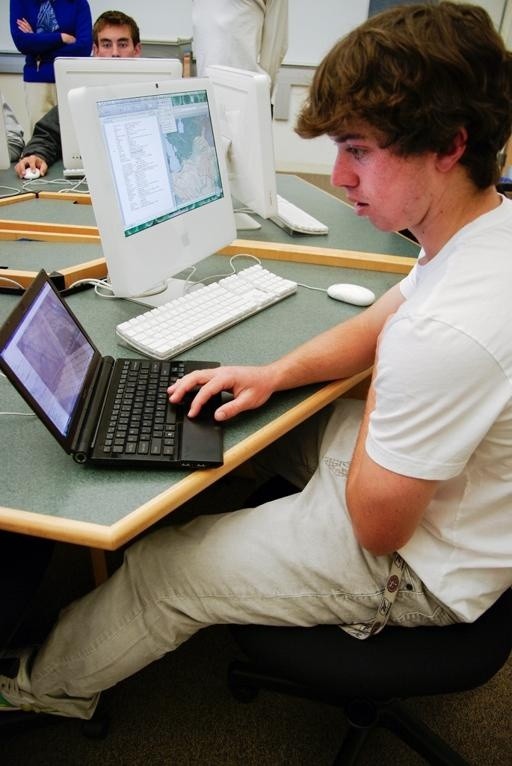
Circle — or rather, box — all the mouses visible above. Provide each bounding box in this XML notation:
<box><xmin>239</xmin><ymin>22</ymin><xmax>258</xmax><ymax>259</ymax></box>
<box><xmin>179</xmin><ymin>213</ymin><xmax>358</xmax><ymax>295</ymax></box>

<box><xmin>327</xmin><ymin>283</ymin><xmax>376</xmax><ymax>307</ymax></box>
<box><xmin>23</xmin><ymin>167</ymin><xmax>40</xmax><ymax>180</ymax></box>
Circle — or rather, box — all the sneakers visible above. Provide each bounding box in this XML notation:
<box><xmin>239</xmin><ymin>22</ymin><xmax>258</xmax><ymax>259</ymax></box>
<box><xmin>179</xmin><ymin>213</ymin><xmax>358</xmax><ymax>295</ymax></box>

<box><xmin>0</xmin><ymin>655</ymin><xmax>39</xmax><ymax>713</ymax></box>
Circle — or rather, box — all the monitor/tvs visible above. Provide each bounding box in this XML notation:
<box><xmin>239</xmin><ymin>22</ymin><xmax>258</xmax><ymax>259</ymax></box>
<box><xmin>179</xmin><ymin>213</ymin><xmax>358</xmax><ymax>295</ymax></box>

<box><xmin>66</xmin><ymin>76</ymin><xmax>238</xmax><ymax>309</ymax></box>
<box><xmin>53</xmin><ymin>57</ymin><xmax>183</xmax><ymax>170</ymax></box>
<box><xmin>204</xmin><ymin>63</ymin><xmax>279</xmax><ymax>231</ymax></box>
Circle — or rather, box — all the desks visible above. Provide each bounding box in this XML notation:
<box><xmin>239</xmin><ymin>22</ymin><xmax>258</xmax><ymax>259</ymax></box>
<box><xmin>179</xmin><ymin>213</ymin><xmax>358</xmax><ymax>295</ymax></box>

<box><xmin>0</xmin><ymin>160</ymin><xmax>511</xmax><ymax>591</ymax></box>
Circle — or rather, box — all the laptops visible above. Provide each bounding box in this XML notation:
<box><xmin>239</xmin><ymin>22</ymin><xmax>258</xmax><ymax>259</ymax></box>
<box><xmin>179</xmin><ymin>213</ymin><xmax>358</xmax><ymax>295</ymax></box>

<box><xmin>0</xmin><ymin>267</ymin><xmax>225</xmax><ymax>471</ymax></box>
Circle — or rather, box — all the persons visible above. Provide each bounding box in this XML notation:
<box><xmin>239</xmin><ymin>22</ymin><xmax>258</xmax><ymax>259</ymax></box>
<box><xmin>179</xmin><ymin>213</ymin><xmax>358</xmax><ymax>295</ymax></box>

<box><xmin>1</xmin><ymin>0</ymin><xmax>512</xmax><ymax>727</ymax></box>
<box><xmin>1</xmin><ymin>96</ymin><xmax>25</xmax><ymax>171</ymax></box>
<box><xmin>14</xmin><ymin>8</ymin><xmax>142</xmax><ymax>182</ymax></box>
<box><xmin>190</xmin><ymin>0</ymin><xmax>289</xmax><ymax>130</ymax></box>
<box><xmin>6</xmin><ymin>0</ymin><xmax>94</xmax><ymax>82</ymax></box>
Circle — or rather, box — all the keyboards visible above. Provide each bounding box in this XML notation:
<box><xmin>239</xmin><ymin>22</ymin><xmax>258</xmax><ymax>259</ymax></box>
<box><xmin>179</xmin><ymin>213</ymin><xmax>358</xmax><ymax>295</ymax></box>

<box><xmin>63</xmin><ymin>169</ymin><xmax>85</xmax><ymax>180</ymax></box>
<box><xmin>115</xmin><ymin>262</ymin><xmax>298</xmax><ymax>360</ymax></box>
<box><xmin>269</xmin><ymin>192</ymin><xmax>330</xmax><ymax>239</ymax></box>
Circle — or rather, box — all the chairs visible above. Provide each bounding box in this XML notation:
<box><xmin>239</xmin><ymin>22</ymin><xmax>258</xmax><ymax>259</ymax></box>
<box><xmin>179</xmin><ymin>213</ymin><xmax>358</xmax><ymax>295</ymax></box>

<box><xmin>220</xmin><ymin>591</ymin><xmax>508</xmax><ymax>765</ymax></box>
